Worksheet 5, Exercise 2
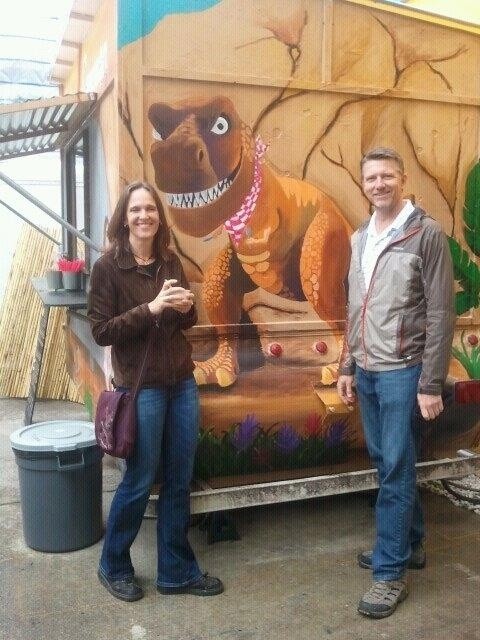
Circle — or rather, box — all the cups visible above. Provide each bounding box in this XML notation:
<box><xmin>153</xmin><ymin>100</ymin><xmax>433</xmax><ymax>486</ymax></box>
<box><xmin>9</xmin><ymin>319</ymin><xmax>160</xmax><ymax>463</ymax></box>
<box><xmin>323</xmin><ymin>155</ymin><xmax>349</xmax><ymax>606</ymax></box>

<box><xmin>44</xmin><ymin>270</ymin><xmax>62</xmax><ymax>290</ymax></box>
<box><xmin>61</xmin><ymin>270</ymin><xmax>83</xmax><ymax>291</ymax></box>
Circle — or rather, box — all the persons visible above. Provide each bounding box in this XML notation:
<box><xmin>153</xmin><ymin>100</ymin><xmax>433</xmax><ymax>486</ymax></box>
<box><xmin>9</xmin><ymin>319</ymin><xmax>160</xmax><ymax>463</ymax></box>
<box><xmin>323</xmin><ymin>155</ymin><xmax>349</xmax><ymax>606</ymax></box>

<box><xmin>335</xmin><ymin>145</ymin><xmax>457</xmax><ymax>620</ymax></box>
<box><xmin>84</xmin><ymin>181</ymin><xmax>225</xmax><ymax>600</ymax></box>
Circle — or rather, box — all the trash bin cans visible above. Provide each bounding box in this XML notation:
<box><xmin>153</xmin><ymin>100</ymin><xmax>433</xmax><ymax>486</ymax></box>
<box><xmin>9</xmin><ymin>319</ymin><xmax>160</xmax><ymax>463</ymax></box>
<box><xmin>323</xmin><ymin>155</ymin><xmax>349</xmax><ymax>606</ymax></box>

<box><xmin>9</xmin><ymin>419</ymin><xmax>104</xmax><ymax>553</ymax></box>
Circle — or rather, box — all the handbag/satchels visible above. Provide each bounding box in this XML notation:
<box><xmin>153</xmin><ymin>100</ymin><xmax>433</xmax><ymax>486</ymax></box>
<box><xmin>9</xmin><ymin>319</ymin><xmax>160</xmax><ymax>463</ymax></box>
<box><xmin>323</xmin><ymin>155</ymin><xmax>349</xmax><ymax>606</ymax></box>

<box><xmin>95</xmin><ymin>392</ymin><xmax>138</xmax><ymax>458</ymax></box>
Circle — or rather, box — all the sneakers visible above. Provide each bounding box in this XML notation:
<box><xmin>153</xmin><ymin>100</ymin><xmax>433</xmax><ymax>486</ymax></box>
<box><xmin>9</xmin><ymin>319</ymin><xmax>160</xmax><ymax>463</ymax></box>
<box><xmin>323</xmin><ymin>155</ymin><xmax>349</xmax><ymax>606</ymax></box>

<box><xmin>357</xmin><ymin>572</ymin><xmax>410</xmax><ymax>618</ymax></box>
<box><xmin>358</xmin><ymin>541</ymin><xmax>425</xmax><ymax>570</ymax></box>
<box><xmin>97</xmin><ymin>562</ymin><xmax>143</xmax><ymax>602</ymax></box>
<box><xmin>157</xmin><ymin>575</ymin><xmax>223</xmax><ymax>596</ymax></box>
